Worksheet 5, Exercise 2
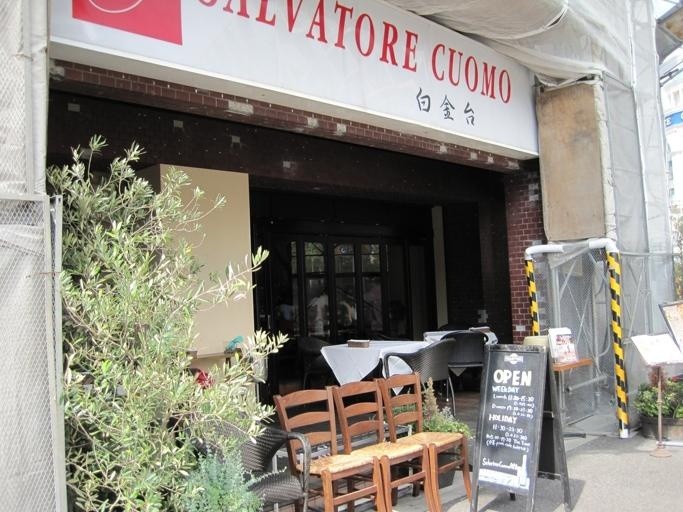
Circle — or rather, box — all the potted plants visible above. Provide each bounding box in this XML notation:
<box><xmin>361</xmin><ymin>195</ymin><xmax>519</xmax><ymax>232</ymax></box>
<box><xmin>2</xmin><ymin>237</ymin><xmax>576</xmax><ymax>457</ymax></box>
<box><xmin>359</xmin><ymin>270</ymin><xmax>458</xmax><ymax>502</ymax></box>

<box><xmin>632</xmin><ymin>377</ymin><xmax>682</xmax><ymax>442</ymax></box>
<box><xmin>382</xmin><ymin>377</ymin><xmax>476</xmax><ymax>490</ymax></box>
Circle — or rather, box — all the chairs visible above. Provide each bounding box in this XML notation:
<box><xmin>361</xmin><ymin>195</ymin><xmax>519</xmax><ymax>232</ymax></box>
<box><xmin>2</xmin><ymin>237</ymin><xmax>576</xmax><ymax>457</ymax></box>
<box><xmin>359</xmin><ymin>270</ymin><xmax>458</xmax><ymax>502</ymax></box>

<box><xmin>438</xmin><ymin>331</ymin><xmax>488</xmax><ymax>402</ymax></box>
<box><xmin>271</xmin><ymin>386</ymin><xmax>386</xmax><ymax>512</ymax></box>
<box><xmin>360</xmin><ymin>330</ymin><xmax>391</xmax><ymax>340</ymax></box>
<box><xmin>329</xmin><ymin>379</ymin><xmax>437</xmax><ymax>512</ymax></box>
<box><xmin>165</xmin><ymin>409</ymin><xmax>310</xmax><ymax>512</ymax></box>
<box><xmin>295</xmin><ymin>335</ymin><xmax>332</xmax><ymax>409</ymax></box>
<box><xmin>383</xmin><ymin>338</ymin><xmax>456</xmax><ymax>417</ymax></box>
<box><xmin>375</xmin><ymin>371</ymin><xmax>471</xmax><ymax>512</ymax></box>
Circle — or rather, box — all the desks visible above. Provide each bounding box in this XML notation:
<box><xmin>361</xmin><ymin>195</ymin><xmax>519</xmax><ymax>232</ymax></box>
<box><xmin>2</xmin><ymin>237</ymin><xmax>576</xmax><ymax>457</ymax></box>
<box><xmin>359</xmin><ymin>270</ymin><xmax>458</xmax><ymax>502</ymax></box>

<box><xmin>319</xmin><ymin>340</ymin><xmax>434</xmax><ymax>396</ymax></box>
<box><xmin>552</xmin><ymin>358</ymin><xmax>592</xmax><ymax>438</ymax></box>
<box><xmin>422</xmin><ymin>331</ymin><xmax>498</xmax><ymax>393</ymax></box>
